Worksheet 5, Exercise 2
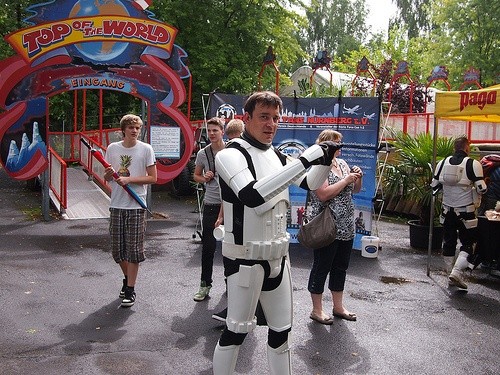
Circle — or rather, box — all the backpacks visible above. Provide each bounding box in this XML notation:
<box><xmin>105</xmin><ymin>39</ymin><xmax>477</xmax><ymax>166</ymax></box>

<box><xmin>479</xmin><ymin>154</ymin><xmax>500</xmax><ymax>184</ymax></box>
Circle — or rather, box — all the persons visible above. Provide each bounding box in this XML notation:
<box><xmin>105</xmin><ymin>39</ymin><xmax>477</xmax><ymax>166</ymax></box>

<box><xmin>431</xmin><ymin>137</ymin><xmax>488</xmax><ymax>290</ymax></box>
<box><xmin>215</xmin><ymin>115</ymin><xmax>247</xmax><ymax>230</ymax></box>
<box><xmin>305</xmin><ymin>127</ymin><xmax>362</xmax><ymax>326</ymax></box>
<box><xmin>214</xmin><ymin>93</ymin><xmax>345</xmax><ymax>375</ymax></box>
<box><xmin>192</xmin><ymin>117</ymin><xmax>231</xmax><ymax>301</ymax></box>
<box><xmin>104</xmin><ymin>114</ymin><xmax>158</xmax><ymax>308</ymax></box>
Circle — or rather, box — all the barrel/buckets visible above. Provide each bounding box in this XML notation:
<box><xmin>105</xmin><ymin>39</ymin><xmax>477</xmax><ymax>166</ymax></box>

<box><xmin>360</xmin><ymin>236</ymin><xmax>379</xmax><ymax>258</ymax></box>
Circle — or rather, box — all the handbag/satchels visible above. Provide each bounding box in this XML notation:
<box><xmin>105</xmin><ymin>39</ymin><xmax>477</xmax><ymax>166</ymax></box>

<box><xmin>296</xmin><ymin>204</ymin><xmax>338</xmax><ymax>251</ymax></box>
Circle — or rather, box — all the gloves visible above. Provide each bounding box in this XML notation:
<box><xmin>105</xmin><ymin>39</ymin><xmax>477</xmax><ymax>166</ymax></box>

<box><xmin>323</xmin><ymin>141</ymin><xmax>345</xmax><ymax>166</ymax></box>
<box><xmin>309</xmin><ymin>143</ymin><xmax>328</xmax><ymax>166</ymax></box>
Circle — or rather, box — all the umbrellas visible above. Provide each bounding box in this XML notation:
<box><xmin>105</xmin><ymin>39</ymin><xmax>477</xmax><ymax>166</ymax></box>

<box><xmin>81</xmin><ymin>137</ymin><xmax>153</xmax><ymax>216</ymax></box>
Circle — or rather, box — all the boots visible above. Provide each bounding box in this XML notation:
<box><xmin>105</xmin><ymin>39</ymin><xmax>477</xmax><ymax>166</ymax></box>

<box><xmin>442</xmin><ymin>256</ymin><xmax>457</xmax><ymax>277</ymax></box>
<box><xmin>449</xmin><ymin>251</ymin><xmax>470</xmax><ymax>289</ymax></box>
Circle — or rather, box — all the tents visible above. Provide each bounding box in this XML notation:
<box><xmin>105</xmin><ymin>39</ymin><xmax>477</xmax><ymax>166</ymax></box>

<box><xmin>426</xmin><ymin>84</ymin><xmax>500</xmax><ymax>277</ymax></box>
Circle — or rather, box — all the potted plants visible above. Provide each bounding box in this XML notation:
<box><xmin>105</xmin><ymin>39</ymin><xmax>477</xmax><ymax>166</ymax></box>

<box><xmin>376</xmin><ymin>127</ymin><xmax>482</xmax><ymax>250</ymax></box>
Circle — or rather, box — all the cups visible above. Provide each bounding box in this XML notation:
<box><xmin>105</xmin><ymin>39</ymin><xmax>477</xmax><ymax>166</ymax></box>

<box><xmin>213</xmin><ymin>225</ymin><xmax>225</xmax><ymax>239</ymax></box>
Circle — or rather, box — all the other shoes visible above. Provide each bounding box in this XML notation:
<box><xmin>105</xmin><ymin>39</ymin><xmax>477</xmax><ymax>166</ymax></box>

<box><xmin>121</xmin><ymin>287</ymin><xmax>136</xmax><ymax>306</ymax></box>
<box><xmin>310</xmin><ymin>310</ymin><xmax>334</xmax><ymax>325</ymax></box>
<box><xmin>212</xmin><ymin>308</ymin><xmax>228</xmax><ymax>323</ymax></box>
<box><xmin>193</xmin><ymin>286</ymin><xmax>210</xmax><ymax>301</ymax></box>
<box><xmin>332</xmin><ymin>309</ymin><xmax>357</xmax><ymax>321</ymax></box>
<box><xmin>119</xmin><ymin>279</ymin><xmax>127</xmax><ymax>298</ymax></box>
<box><xmin>481</xmin><ymin>264</ymin><xmax>500</xmax><ymax>277</ymax></box>
